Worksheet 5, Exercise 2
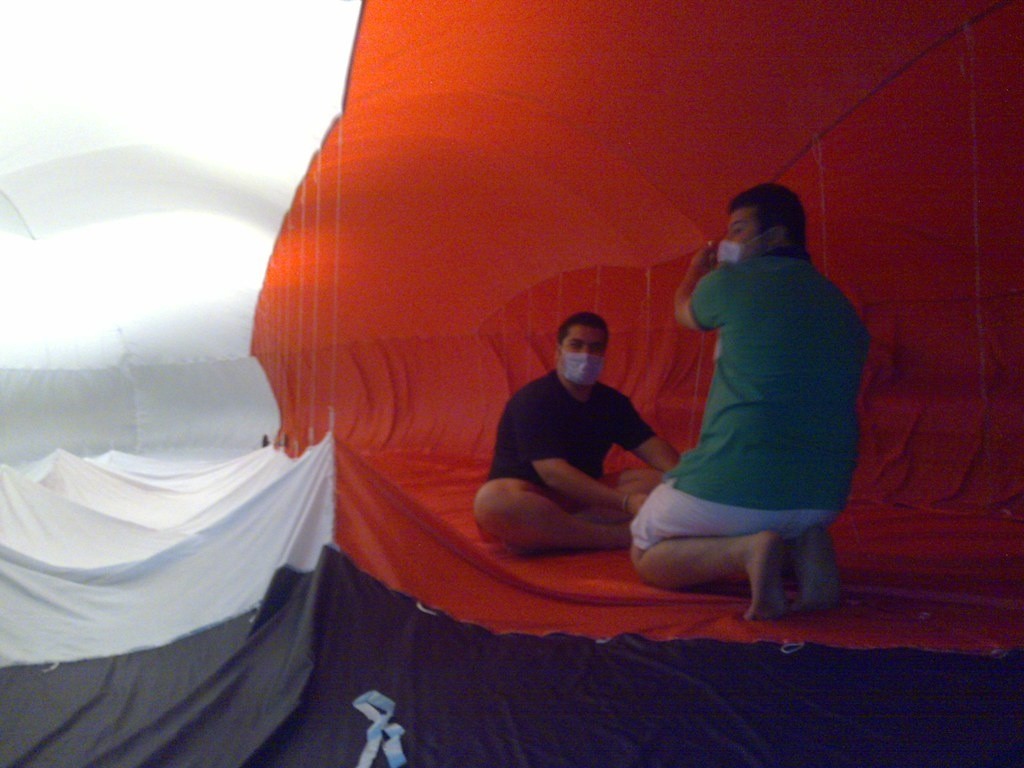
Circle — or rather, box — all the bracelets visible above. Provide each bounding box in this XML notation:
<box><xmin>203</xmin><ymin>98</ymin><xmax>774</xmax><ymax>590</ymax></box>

<box><xmin>623</xmin><ymin>493</ymin><xmax>631</xmax><ymax>514</ymax></box>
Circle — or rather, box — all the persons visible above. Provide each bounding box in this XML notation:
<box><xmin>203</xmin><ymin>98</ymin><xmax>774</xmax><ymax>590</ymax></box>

<box><xmin>629</xmin><ymin>183</ymin><xmax>871</xmax><ymax>618</ymax></box>
<box><xmin>473</xmin><ymin>310</ymin><xmax>680</xmax><ymax>557</ymax></box>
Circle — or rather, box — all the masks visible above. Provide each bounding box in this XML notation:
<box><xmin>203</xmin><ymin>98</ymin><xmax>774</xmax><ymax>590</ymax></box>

<box><xmin>557</xmin><ymin>347</ymin><xmax>606</xmax><ymax>385</ymax></box>
<box><xmin>718</xmin><ymin>226</ymin><xmax>778</xmax><ymax>265</ymax></box>
